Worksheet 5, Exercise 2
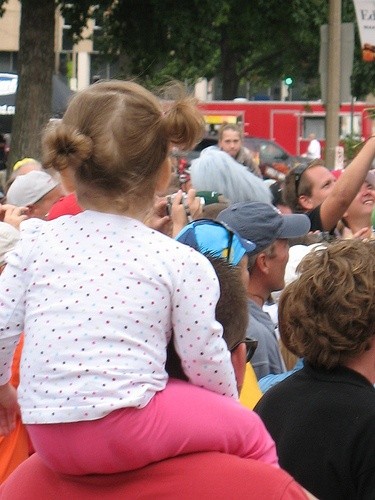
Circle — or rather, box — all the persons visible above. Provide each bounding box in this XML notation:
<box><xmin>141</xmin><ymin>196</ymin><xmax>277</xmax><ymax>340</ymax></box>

<box><xmin>252</xmin><ymin>238</ymin><xmax>375</xmax><ymax>500</ymax></box>
<box><xmin>0</xmin><ymin>80</ymin><xmax>279</xmax><ymax>476</ymax></box>
<box><xmin>0</xmin><ymin>331</ymin><xmax>35</xmax><ymax>484</ymax></box>
<box><xmin>0</xmin><ymin>124</ymin><xmax>375</xmax><ymax>410</ymax></box>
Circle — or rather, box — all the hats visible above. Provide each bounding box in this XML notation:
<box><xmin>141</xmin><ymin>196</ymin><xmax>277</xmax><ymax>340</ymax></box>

<box><xmin>175</xmin><ymin>218</ymin><xmax>256</xmax><ymax>266</ymax></box>
<box><xmin>6</xmin><ymin>170</ymin><xmax>57</xmax><ymax>207</ymax></box>
<box><xmin>216</xmin><ymin>200</ymin><xmax>311</xmax><ymax>257</ymax></box>
<box><xmin>195</xmin><ymin>191</ymin><xmax>230</xmax><ymax>222</ymax></box>
<box><xmin>13</xmin><ymin>157</ymin><xmax>35</xmax><ymax>172</ymax></box>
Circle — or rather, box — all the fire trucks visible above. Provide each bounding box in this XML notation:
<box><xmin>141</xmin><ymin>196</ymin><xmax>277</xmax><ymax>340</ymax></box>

<box><xmin>196</xmin><ymin>98</ymin><xmax>375</xmax><ymax>163</ymax></box>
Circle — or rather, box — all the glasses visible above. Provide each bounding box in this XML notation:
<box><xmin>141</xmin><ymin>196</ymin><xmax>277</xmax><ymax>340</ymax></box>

<box><xmin>230</xmin><ymin>337</ymin><xmax>258</xmax><ymax>363</ymax></box>
<box><xmin>291</xmin><ymin>161</ymin><xmax>313</xmax><ymax>199</ymax></box>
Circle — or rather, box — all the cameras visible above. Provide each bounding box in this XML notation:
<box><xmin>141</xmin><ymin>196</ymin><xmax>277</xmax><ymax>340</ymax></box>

<box><xmin>166</xmin><ymin>192</ymin><xmax>205</xmax><ymax>217</ymax></box>
